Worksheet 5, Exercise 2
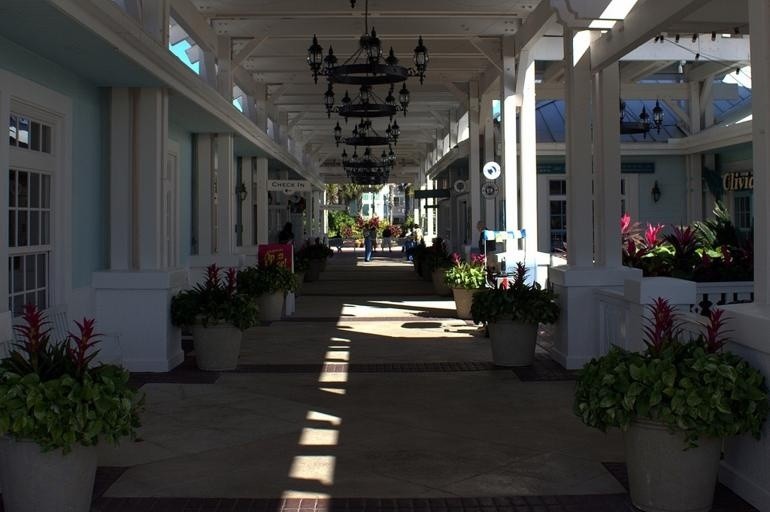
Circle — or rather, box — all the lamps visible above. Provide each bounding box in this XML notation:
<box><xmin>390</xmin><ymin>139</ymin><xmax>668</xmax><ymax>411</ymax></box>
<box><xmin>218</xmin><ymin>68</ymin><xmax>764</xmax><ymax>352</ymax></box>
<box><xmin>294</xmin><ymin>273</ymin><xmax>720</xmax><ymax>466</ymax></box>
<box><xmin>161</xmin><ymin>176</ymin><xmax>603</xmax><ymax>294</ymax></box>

<box><xmin>303</xmin><ymin>1</ymin><xmax>431</xmax><ymax>188</ymax></box>
<box><xmin>269</xmin><ymin>190</ymin><xmax>272</xmax><ymax>206</ymax></box>
<box><xmin>619</xmin><ymin>96</ymin><xmax>666</xmax><ymax>140</ymax></box>
<box><xmin>235</xmin><ymin>184</ymin><xmax>247</xmax><ymax>200</ymax></box>
<box><xmin>650</xmin><ymin>179</ymin><xmax>662</xmax><ymax>204</ymax></box>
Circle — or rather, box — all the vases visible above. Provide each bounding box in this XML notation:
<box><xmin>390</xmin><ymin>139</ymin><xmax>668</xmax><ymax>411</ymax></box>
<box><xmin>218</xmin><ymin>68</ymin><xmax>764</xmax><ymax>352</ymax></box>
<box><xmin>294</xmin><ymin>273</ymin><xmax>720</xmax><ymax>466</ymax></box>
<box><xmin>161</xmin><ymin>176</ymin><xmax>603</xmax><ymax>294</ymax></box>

<box><xmin>192</xmin><ymin>320</ymin><xmax>241</xmax><ymax>371</ymax></box>
<box><xmin>431</xmin><ymin>270</ymin><xmax>451</xmax><ymax>297</ymax></box>
<box><xmin>415</xmin><ymin>256</ymin><xmax>426</xmax><ymax>274</ymax></box>
<box><xmin>453</xmin><ymin>284</ymin><xmax>493</xmax><ymax>323</ymax></box>
<box><xmin>253</xmin><ymin>293</ymin><xmax>284</xmax><ymax>321</ymax></box>
<box><xmin>420</xmin><ymin>263</ymin><xmax>433</xmax><ymax>281</ymax></box>
<box><xmin>623</xmin><ymin>417</ymin><xmax>721</xmax><ymax>511</ymax></box>
<box><xmin>1</xmin><ymin>438</ymin><xmax>98</xmax><ymax>512</ymax></box>
<box><xmin>486</xmin><ymin>319</ymin><xmax>539</xmax><ymax>368</ymax></box>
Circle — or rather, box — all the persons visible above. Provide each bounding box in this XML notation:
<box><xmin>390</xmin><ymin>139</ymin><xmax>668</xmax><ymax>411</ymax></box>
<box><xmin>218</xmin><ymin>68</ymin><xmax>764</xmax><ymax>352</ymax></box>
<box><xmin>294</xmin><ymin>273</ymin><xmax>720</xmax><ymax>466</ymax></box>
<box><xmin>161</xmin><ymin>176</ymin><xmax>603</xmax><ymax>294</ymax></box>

<box><xmin>476</xmin><ymin>220</ymin><xmax>493</xmax><ymax>256</ymax></box>
<box><xmin>275</xmin><ymin>222</ymin><xmax>294</xmax><ymax>244</ymax></box>
<box><xmin>328</xmin><ymin>232</ymin><xmax>343</xmax><ymax>254</ymax></box>
<box><xmin>364</xmin><ymin>221</ymin><xmax>421</xmax><ymax>262</ymax></box>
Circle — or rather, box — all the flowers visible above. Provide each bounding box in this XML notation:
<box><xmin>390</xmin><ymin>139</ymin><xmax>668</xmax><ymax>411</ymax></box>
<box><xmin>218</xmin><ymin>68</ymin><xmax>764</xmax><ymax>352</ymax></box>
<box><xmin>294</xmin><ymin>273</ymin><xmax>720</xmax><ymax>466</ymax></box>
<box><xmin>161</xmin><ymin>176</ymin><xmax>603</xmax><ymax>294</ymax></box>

<box><xmin>569</xmin><ymin>295</ymin><xmax>770</xmax><ymax>442</ymax></box>
<box><xmin>168</xmin><ymin>255</ymin><xmax>260</xmax><ymax>330</ymax></box>
<box><xmin>441</xmin><ymin>250</ymin><xmax>490</xmax><ymax>288</ymax></box>
<box><xmin>427</xmin><ymin>237</ymin><xmax>447</xmax><ymax>257</ymax></box>
<box><xmin>235</xmin><ymin>251</ymin><xmax>298</xmax><ymax>296</ymax></box>
<box><xmin>0</xmin><ymin>300</ymin><xmax>148</xmax><ymax>457</ymax></box>
<box><xmin>468</xmin><ymin>263</ymin><xmax>562</xmax><ymax>327</ymax></box>
<box><xmin>340</xmin><ymin>215</ymin><xmax>401</xmax><ymax>239</ymax></box>
<box><xmin>619</xmin><ymin>206</ymin><xmax>754</xmax><ymax>273</ymax></box>
